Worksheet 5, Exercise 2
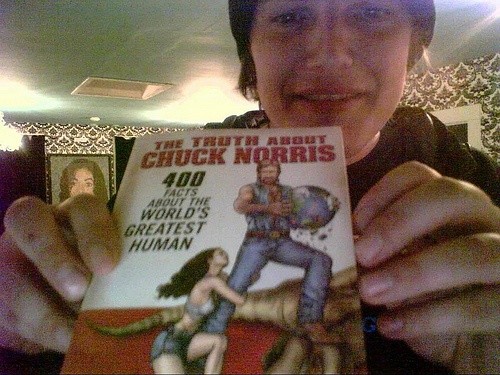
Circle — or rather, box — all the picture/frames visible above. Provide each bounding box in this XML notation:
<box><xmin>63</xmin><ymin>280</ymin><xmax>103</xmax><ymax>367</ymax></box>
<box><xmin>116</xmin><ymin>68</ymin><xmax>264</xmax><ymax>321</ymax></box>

<box><xmin>428</xmin><ymin>104</ymin><xmax>484</xmax><ymax>154</ymax></box>
<box><xmin>46</xmin><ymin>152</ymin><xmax>114</xmax><ymax>208</ymax></box>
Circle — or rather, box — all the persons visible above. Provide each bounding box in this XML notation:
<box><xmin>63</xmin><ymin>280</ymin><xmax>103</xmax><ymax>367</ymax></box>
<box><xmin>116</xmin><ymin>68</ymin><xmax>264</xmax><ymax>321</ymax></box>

<box><xmin>0</xmin><ymin>0</ymin><xmax>500</xmax><ymax>375</ymax></box>
<box><xmin>59</xmin><ymin>158</ymin><xmax>106</xmax><ymax>204</ymax></box>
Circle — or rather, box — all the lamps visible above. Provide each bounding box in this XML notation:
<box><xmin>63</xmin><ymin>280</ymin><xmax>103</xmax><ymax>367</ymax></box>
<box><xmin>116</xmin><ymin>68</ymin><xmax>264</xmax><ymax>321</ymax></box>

<box><xmin>0</xmin><ymin>111</ymin><xmax>24</xmax><ymax>153</ymax></box>
